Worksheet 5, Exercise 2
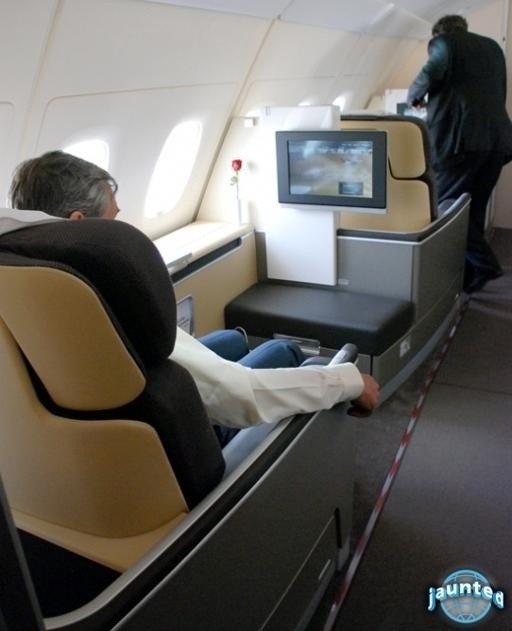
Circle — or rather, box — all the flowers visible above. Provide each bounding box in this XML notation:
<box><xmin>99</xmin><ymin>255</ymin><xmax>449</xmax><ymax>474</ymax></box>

<box><xmin>230</xmin><ymin>159</ymin><xmax>243</xmax><ymax>199</ymax></box>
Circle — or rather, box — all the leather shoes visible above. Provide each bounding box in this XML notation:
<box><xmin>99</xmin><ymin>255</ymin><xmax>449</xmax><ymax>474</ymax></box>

<box><xmin>465</xmin><ymin>267</ymin><xmax>504</xmax><ymax>294</ymax></box>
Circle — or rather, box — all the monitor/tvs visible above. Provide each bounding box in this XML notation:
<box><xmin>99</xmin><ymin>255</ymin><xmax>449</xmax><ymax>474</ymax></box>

<box><xmin>275</xmin><ymin>129</ymin><xmax>390</xmax><ymax>215</ymax></box>
<box><xmin>396</xmin><ymin>104</ymin><xmax>427</xmax><ymax>121</ymax></box>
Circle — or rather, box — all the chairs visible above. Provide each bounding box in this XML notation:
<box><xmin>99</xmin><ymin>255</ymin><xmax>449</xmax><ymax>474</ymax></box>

<box><xmin>332</xmin><ymin>109</ymin><xmax>473</xmax><ymax>390</ymax></box>
<box><xmin>1</xmin><ymin>214</ymin><xmax>371</xmax><ymax>629</ymax></box>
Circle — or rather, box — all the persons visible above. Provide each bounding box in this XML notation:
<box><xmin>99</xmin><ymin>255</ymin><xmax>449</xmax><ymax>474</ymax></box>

<box><xmin>0</xmin><ymin>148</ymin><xmax>383</xmax><ymax>451</ymax></box>
<box><xmin>406</xmin><ymin>13</ymin><xmax>511</xmax><ymax>295</ymax></box>
